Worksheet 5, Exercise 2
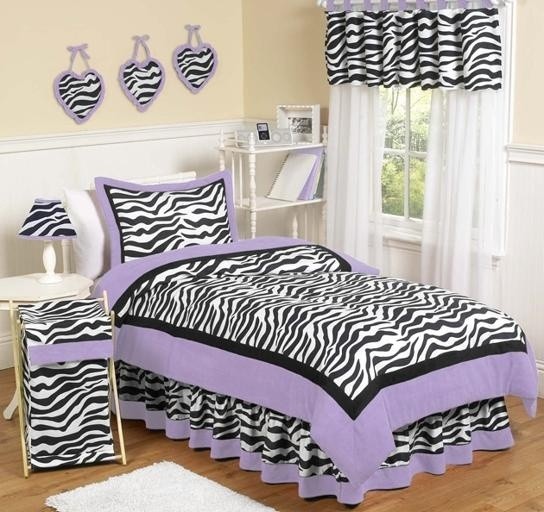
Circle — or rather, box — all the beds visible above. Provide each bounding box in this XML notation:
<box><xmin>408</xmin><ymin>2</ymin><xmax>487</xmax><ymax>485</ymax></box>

<box><xmin>57</xmin><ymin>168</ymin><xmax>538</xmax><ymax>505</ymax></box>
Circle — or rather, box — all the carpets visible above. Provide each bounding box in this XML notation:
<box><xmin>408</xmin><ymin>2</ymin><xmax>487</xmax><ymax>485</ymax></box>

<box><xmin>43</xmin><ymin>459</ymin><xmax>282</xmax><ymax>512</ymax></box>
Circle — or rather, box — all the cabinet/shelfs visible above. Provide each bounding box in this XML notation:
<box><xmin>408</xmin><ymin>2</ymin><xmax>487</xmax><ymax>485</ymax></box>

<box><xmin>211</xmin><ymin>127</ymin><xmax>327</xmax><ymax>241</ymax></box>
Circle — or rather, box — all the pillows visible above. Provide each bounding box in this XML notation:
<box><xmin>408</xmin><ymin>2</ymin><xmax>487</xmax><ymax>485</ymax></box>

<box><xmin>60</xmin><ymin>171</ymin><xmax>196</xmax><ymax>279</ymax></box>
<box><xmin>93</xmin><ymin>169</ymin><xmax>238</xmax><ymax>270</ymax></box>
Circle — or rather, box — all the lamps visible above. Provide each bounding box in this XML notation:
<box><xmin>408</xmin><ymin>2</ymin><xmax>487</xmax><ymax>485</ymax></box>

<box><xmin>17</xmin><ymin>197</ymin><xmax>78</xmax><ymax>284</ymax></box>
